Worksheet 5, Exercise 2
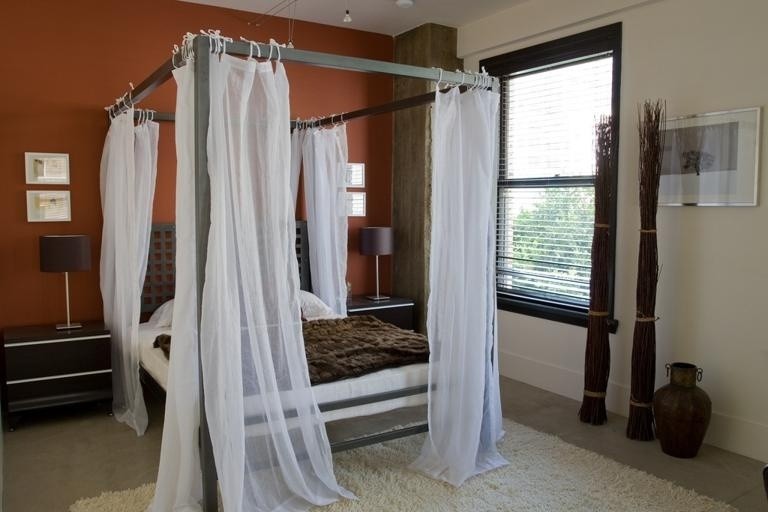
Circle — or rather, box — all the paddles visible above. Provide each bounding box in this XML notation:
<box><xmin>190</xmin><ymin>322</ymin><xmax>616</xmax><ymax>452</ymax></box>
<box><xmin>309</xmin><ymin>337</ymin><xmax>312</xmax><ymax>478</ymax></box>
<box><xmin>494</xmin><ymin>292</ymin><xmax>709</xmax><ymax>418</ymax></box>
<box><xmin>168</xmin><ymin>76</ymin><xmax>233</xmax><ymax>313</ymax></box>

<box><xmin>65</xmin><ymin>415</ymin><xmax>743</xmax><ymax>512</ymax></box>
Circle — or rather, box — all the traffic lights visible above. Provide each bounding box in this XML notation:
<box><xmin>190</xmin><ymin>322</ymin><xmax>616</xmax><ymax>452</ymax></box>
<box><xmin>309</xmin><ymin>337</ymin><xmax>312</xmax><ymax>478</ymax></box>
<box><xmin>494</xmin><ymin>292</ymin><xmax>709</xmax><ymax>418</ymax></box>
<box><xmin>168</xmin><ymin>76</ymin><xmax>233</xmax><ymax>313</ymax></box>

<box><xmin>148</xmin><ymin>290</ymin><xmax>337</xmax><ymax>331</ymax></box>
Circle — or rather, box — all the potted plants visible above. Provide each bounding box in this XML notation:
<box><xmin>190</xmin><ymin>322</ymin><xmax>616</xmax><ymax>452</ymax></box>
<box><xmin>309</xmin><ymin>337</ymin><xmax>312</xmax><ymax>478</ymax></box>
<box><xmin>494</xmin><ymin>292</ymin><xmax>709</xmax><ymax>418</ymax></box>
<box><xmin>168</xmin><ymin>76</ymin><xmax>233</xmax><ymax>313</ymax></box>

<box><xmin>336</xmin><ymin>162</ymin><xmax>366</xmax><ymax>189</ymax></box>
<box><xmin>24</xmin><ymin>151</ymin><xmax>71</xmax><ymax>185</ymax></box>
<box><xmin>640</xmin><ymin>106</ymin><xmax>764</xmax><ymax>207</ymax></box>
<box><xmin>26</xmin><ymin>190</ymin><xmax>72</xmax><ymax>223</ymax></box>
<box><xmin>338</xmin><ymin>192</ymin><xmax>367</xmax><ymax>217</ymax></box>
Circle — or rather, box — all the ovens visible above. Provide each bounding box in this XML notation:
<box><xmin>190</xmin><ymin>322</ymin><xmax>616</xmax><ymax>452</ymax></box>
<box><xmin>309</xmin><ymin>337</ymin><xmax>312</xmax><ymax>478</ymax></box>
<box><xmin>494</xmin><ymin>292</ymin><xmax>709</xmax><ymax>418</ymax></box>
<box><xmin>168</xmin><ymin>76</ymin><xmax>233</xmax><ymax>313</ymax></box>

<box><xmin>138</xmin><ymin>220</ymin><xmax>442</xmax><ymax>481</ymax></box>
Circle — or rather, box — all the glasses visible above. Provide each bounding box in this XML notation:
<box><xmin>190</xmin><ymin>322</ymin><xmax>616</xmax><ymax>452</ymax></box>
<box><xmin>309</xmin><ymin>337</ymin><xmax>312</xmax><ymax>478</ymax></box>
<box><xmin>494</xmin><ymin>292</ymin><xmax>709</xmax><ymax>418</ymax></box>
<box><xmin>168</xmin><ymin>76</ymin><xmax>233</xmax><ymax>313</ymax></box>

<box><xmin>344</xmin><ymin>9</ymin><xmax>353</xmax><ymax>22</ymax></box>
<box><xmin>287</xmin><ymin>0</ymin><xmax>296</xmax><ymax>49</ymax></box>
<box><xmin>39</xmin><ymin>234</ymin><xmax>93</xmax><ymax>330</ymax></box>
<box><xmin>395</xmin><ymin>0</ymin><xmax>414</xmax><ymax>9</ymax></box>
<box><xmin>359</xmin><ymin>225</ymin><xmax>395</xmax><ymax>301</ymax></box>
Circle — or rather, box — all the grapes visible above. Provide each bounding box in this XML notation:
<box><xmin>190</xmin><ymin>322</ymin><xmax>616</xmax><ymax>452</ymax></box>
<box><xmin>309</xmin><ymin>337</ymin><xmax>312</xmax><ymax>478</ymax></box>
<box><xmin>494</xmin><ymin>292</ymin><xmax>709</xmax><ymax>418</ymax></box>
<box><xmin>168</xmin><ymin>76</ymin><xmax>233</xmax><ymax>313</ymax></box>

<box><xmin>346</xmin><ymin>292</ymin><xmax>416</xmax><ymax>330</ymax></box>
<box><xmin>1</xmin><ymin>321</ymin><xmax>114</xmax><ymax>432</ymax></box>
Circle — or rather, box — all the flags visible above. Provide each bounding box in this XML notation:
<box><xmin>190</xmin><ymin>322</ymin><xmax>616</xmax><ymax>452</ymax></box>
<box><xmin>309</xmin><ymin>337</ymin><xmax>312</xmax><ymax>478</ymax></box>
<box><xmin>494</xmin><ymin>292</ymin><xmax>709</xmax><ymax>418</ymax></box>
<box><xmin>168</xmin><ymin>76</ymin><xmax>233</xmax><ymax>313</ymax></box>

<box><xmin>653</xmin><ymin>362</ymin><xmax>712</xmax><ymax>459</ymax></box>
